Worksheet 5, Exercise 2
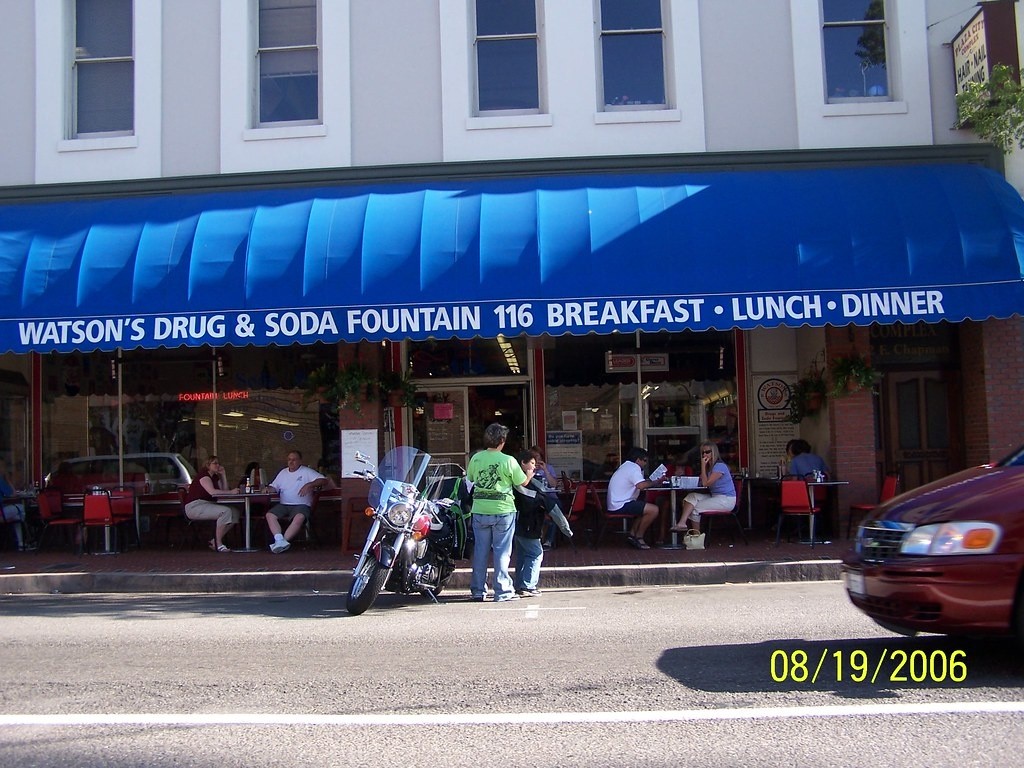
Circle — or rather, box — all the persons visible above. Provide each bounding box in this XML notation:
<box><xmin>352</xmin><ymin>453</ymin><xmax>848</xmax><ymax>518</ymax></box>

<box><xmin>669</xmin><ymin>441</ymin><xmax>737</xmax><ymax>534</ymax></box>
<box><xmin>0</xmin><ymin>472</ymin><xmax>37</xmax><ymax>551</ymax></box>
<box><xmin>261</xmin><ymin>451</ymin><xmax>327</xmax><ymax>554</ymax></box>
<box><xmin>606</xmin><ymin>447</ymin><xmax>659</xmax><ymax>549</ymax></box>
<box><xmin>779</xmin><ymin>439</ymin><xmax>832</xmax><ymax>504</ymax></box>
<box><xmin>239</xmin><ymin>461</ymin><xmax>261</xmax><ymax>487</ymax></box>
<box><xmin>184</xmin><ymin>455</ymin><xmax>240</xmax><ymax>552</ymax></box>
<box><xmin>181</xmin><ymin>436</ymin><xmax>208</xmax><ymax>470</ymax></box>
<box><xmin>315</xmin><ymin>458</ymin><xmax>337</xmax><ymax>491</ymax></box>
<box><xmin>466</xmin><ymin>422</ymin><xmax>562</xmax><ymax>601</ymax></box>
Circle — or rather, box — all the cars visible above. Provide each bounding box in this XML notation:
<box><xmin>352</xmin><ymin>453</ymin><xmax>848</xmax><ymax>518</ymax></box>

<box><xmin>840</xmin><ymin>444</ymin><xmax>1024</xmax><ymax>682</ymax></box>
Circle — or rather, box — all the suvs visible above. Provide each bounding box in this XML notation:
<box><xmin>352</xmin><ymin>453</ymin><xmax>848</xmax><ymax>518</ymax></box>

<box><xmin>45</xmin><ymin>450</ymin><xmax>197</xmax><ymax>494</ymax></box>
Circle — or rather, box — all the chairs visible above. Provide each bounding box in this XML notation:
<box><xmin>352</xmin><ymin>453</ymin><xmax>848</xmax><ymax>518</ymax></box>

<box><xmin>146</xmin><ymin>463</ymin><xmax>174</xmax><ymax>473</ymax></box>
<box><xmin>591</xmin><ymin>465</ymin><xmax>693</xmax><ymax>552</ymax></box>
<box><xmin>35</xmin><ymin>487</ymin><xmax>86</xmax><ymax>555</ymax></box>
<box><xmin>0</xmin><ymin>503</ymin><xmax>26</xmax><ymax>553</ymax></box>
<box><xmin>240</xmin><ymin>485</ymin><xmax>268</xmax><ymax>541</ymax></box>
<box><xmin>544</xmin><ymin>482</ymin><xmax>593</xmax><ymax>554</ymax></box>
<box><xmin>80</xmin><ymin>485</ymin><xmax>143</xmax><ymax>557</ymax></box>
<box><xmin>702</xmin><ymin>479</ymin><xmax>749</xmax><ymax>546</ymax></box>
<box><xmin>154</xmin><ymin>484</ymin><xmax>237</xmax><ymax>553</ymax></box>
<box><xmin>279</xmin><ymin>485</ymin><xmax>322</xmax><ymax>547</ymax></box>
<box><xmin>848</xmin><ymin>476</ymin><xmax>897</xmax><ymax>540</ymax></box>
<box><xmin>775</xmin><ymin>472</ymin><xmax>829</xmax><ymax>550</ymax></box>
<box><xmin>341</xmin><ymin>497</ymin><xmax>376</xmax><ymax>555</ymax></box>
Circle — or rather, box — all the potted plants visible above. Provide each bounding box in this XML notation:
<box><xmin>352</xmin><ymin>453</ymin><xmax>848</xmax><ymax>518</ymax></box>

<box><xmin>302</xmin><ymin>359</ymin><xmax>424</xmax><ymax>420</ymax></box>
<box><xmin>783</xmin><ymin>375</ymin><xmax>828</xmax><ymax>424</ymax></box>
<box><xmin>433</xmin><ymin>393</ymin><xmax>456</xmax><ymax>419</ymax></box>
<box><xmin>825</xmin><ymin>353</ymin><xmax>880</xmax><ymax>397</ymax></box>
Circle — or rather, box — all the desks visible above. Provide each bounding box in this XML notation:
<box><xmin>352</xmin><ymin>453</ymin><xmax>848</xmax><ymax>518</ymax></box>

<box><xmin>212</xmin><ymin>494</ymin><xmax>271</xmax><ymax>553</ymax></box>
<box><xmin>68</xmin><ymin>497</ymin><xmax>125</xmax><ymax>555</ymax></box>
<box><xmin>796</xmin><ymin>481</ymin><xmax>850</xmax><ymax>547</ymax></box>
<box><xmin>643</xmin><ymin>487</ymin><xmax>708</xmax><ymax>549</ymax></box>
<box><xmin>745</xmin><ymin>477</ymin><xmax>778</xmax><ymax>529</ymax></box>
<box><xmin>3</xmin><ymin>496</ymin><xmax>37</xmax><ymax>543</ymax></box>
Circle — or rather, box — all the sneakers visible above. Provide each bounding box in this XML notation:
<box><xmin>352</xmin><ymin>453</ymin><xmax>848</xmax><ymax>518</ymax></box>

<box><xmin>269</xmin><ymin>540</ymin><xmax>290</xmax><ymax>554</ymax></box>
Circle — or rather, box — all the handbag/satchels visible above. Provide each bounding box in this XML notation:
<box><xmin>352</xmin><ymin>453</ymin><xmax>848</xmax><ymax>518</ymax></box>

<box><xmin>421</xmin><ymin>478</ymin><xmax>475</xmax><ymax>514</ymax></box>
<box><xmin>441</xmin><ymin>501</ymin><xmax>467</xmax><ymax>560</ymax></box>
<box><xmin>684</xmin><ymin>529</ymin><xmax>705</xmax><ymax>550</ymax></box>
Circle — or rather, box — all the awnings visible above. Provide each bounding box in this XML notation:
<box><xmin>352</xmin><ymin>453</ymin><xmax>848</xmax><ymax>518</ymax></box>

<box><xmin>0</xmin><ymin>166</ymin><xmax>1024</xmax><ymax>357</ymax></box>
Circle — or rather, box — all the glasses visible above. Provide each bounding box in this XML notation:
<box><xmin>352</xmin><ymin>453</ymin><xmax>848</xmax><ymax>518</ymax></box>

<box><xmin>211</xmin><ymin>462</ymin><xmax>220</xmax><ymax>465</ymax></box>
<box><xmin>702</xmin><ymin>450</ymin><xmax>712</xmax><ymax>454</ymax></box>
<box><xmin>642</xmin><ymin>459</ymin><xmax>646</xmax><ymax>462</ymax></box>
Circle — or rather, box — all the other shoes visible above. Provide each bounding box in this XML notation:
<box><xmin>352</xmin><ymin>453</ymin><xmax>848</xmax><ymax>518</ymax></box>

<box><xmin>670</xmin><ymin>524</ymin><xmax>688</xmax><ymax>532</ymax></box>
<box><xmin>217</xmin><ymin>545</ymin><xmax>230</xmax><ymax>553</ymax></box>
<box><xmin>208</xmin><ymin>539</ymin><xmax>216</xmax><ymax>551</ymax></box>
<box><xmin>494</xmin><ymin>594</ymin><xmax>520</xmax><ymax>602</ymax></box>
<box><xmin>469</xmin><ymin>595</ymin><xmax>483</xmax><ymax>602</ymax></box>
<box><xmin>542</xmin><ymin>540</ymin><xmax>552</xmax><ymax>552</ymax></box>
<box><xmin>628</xmin><ymin>534</ymin><xmax>650</xmax><ymax>550</ymax></box>
<box><xmin>18</xmin><ymin>543</ymin><xmax>38</xmax><ymax>551</ymax></box>
<box><xmin>516</xmin><ymin>588</ymin><xmax>542</xmax><ymax>597</ymax></box>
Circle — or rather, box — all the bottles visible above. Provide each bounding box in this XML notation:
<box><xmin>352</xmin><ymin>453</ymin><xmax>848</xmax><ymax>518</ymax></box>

<box><xmin>143</xmin><ymin>478</ymin><xmax>150</xmax><ymax>494</ymax></box>
<box><xmin>812</xmin><ymin>470</ymin><xmax>823</xmax><ymax>483</ymax></box>
<box><xmin>245</xmin><ymin>478</ymin><xmax>251</xmax><ymax>494</ymax></box>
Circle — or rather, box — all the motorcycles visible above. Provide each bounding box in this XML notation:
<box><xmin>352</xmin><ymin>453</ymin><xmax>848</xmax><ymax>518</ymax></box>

<box><xmin>345</xmin><ymin>445</ymin><xmax>471</xmax><ymax>616</ymax></box>
